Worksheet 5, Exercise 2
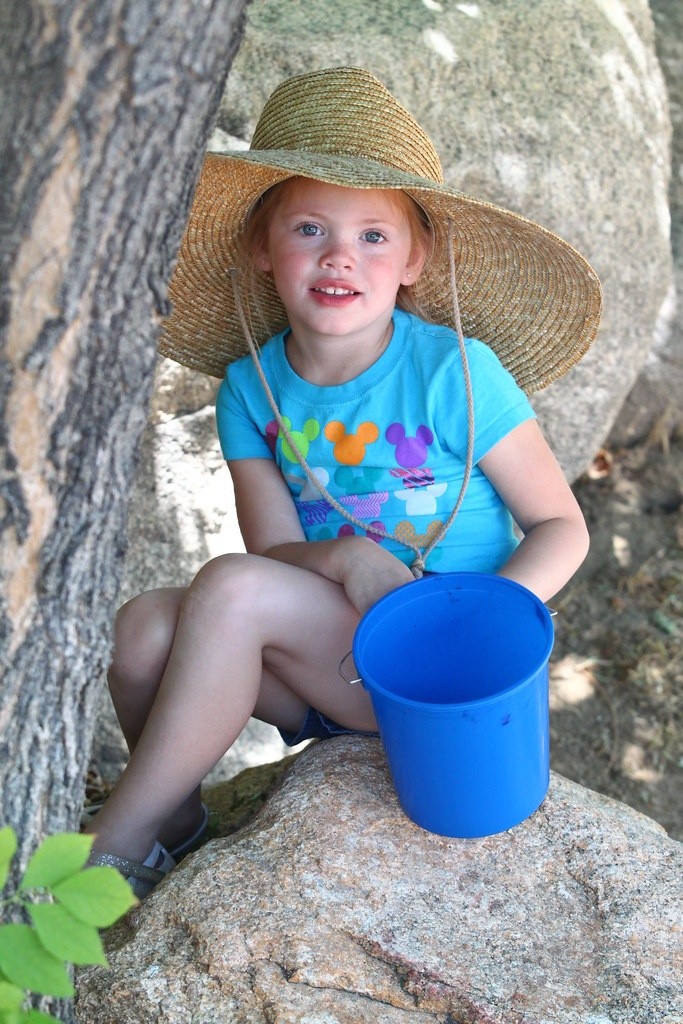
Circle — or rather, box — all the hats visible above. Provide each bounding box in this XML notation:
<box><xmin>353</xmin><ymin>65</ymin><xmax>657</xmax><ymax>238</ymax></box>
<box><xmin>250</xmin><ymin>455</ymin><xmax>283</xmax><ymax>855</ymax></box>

<box><xmin>159</xmin><ymin>65</ymin><xmax>608</xmax><ymax>398</ymax></box>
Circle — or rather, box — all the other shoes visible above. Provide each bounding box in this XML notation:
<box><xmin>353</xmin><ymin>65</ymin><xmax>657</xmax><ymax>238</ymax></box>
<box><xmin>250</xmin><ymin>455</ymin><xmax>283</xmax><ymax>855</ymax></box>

<box><xmin>170</xmin><ymin>803</ymin><xmax>213</xmax><ymax>866</ymax></box>
<box><xmin>83</xmin><ymin>837</ymin><xmax>177</xmax><ymax>917</ymax></box>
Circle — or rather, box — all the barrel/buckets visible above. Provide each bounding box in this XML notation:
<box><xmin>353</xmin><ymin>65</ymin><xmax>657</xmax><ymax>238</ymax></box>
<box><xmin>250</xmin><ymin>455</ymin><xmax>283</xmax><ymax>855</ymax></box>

<box><xmin>336</xmin><ymin>572</ymin><xmax>558</xmax><ymax>839</ymax></box>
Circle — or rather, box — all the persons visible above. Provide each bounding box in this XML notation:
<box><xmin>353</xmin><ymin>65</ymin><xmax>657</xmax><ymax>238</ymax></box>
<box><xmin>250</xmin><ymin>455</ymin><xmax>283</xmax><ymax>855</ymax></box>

<box><xmin>81</xmin><ymin>68</ymin><xmax>602</xmax><ymax>902</ymax></box>
